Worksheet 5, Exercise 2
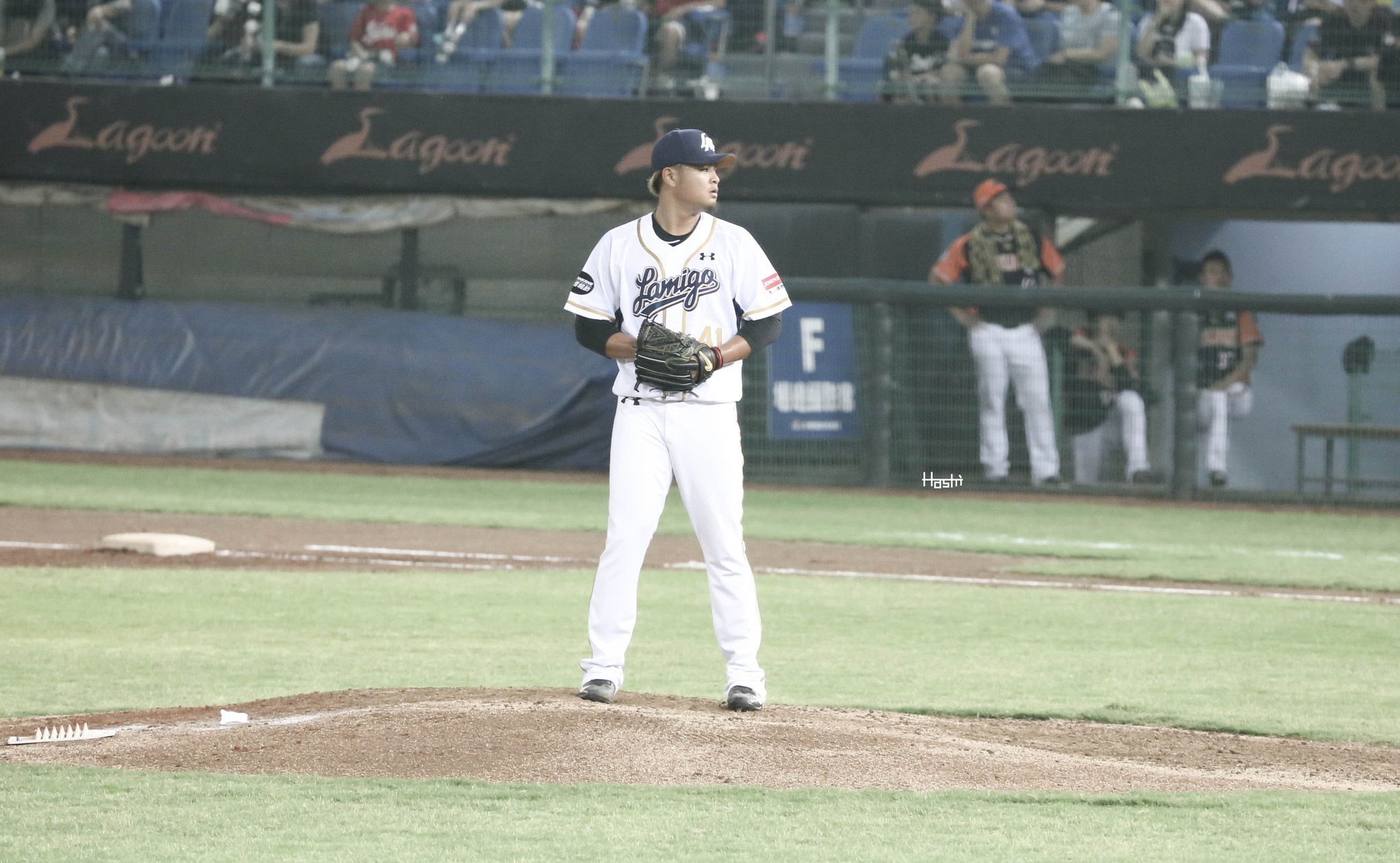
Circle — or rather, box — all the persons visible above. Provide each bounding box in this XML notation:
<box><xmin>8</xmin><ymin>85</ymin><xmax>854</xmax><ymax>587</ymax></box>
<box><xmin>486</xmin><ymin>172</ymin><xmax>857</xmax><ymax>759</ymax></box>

<box><xmin>928</xmin><ymin>178</ymin><xmax>1264</xmax><ymax>485</ymax></box>
<box><xmin>0</xmin><ymin>0</ymin><xmax>1400</xmax><ymax>114</ymax></box>
<box><xmin>563</xmin><ymin>128</ymin><xmax>793</xmax><ymax>712</ymax></box>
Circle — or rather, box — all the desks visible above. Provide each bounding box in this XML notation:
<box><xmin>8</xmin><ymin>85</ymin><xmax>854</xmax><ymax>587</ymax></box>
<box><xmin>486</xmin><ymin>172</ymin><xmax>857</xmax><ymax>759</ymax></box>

<box><xmin>1291</xmin><ymin>420</ymin><xmax>1400</xmax><ymax>511</ymax></box>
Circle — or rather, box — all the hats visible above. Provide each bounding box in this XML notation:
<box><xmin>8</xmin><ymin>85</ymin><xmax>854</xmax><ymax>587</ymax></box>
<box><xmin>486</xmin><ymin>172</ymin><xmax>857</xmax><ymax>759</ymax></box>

<box><xmin>973</xmin><ymin>179</ymin><xmax>1007</xmax><ymax>211</ymax></box>
<box><xmin>652</xmin><ymin>128</ymin><xmax>737</xmax><ymax>171</ymax></box>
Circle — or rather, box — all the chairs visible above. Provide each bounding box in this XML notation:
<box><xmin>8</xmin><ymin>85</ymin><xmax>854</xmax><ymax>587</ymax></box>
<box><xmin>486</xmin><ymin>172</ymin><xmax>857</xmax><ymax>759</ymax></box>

<box><xmin>0</xmin><ymin>0</ymin><xmax>1400</xmax><ymax>127</ymax></box>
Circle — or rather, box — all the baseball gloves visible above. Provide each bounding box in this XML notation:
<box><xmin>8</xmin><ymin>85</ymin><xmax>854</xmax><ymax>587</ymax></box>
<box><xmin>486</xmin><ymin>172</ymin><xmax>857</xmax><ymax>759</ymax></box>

<box><xmin>632</xmin><ymin>318</ymin><xmax>718</xmax><ymax>394</ymax></box>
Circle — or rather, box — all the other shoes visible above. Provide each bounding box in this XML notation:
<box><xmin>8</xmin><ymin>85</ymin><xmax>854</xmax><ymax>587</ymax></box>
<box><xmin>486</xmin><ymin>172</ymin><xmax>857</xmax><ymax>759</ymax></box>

<box><xmin>1043</xmin><ymin>476</ymin><xmax>1066</xmax><ymax>486</ymax></box>
<box><xmin>692</xmin><ymin>79</ymin><xmax>710</xmax><ymax>98</ymax></box>
<box><xmin>1132</xmin><ymin>471</ymin><xmax>1153</xmax><ymax>485</ymax></box>
<box><xmin>1210</xmin><ymin>471</ymin><xmax>1226</xmax><ymax>485</ymax></box>
<box><xmin>654</xmin><ymin>71</ymin><xmax>676</xmax><ymax>93</ymax></box>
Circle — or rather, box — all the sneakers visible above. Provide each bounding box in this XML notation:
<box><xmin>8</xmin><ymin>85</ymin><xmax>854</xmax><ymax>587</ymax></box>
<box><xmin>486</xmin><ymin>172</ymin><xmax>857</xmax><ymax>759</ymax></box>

<box><xmin>579</xmin><ymin>680</ymin><xmax>615</xmax><ymax>705</ymax></box>
<box><xmin>727</xmin><ymin>686</ymin><xmax>762</xmax><ymax>710</ymax></box>
